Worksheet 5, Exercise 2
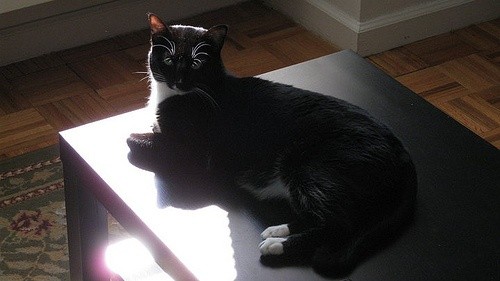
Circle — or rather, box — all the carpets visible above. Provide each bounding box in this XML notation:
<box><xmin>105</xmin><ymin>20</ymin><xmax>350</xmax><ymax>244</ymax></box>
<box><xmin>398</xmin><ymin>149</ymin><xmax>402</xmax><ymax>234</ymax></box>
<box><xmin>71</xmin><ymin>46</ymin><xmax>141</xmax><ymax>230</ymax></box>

<box><xmin>0</xmin><ymin>144</ymin><xmax>70</xmax><ymax>281</ymax></box>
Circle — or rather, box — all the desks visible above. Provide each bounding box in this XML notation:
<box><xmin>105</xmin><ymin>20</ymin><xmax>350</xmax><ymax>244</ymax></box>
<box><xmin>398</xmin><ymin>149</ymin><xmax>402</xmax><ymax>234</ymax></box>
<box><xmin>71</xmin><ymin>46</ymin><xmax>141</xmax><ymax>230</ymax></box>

<box><xmin>57</xmin><ymin>48</ymin><xmax>500</xmax><ymax>280</ymax></box>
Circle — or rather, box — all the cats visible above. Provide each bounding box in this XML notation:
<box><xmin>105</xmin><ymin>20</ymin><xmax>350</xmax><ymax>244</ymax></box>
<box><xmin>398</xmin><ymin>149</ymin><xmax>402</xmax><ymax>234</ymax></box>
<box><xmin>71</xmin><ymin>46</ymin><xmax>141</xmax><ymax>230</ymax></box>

<box><xmin>126</xmin><ymin>11</ymin><xmax>418</xmax><ymax>266</ymax></box>
